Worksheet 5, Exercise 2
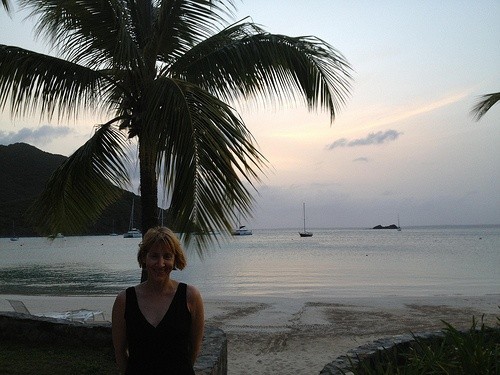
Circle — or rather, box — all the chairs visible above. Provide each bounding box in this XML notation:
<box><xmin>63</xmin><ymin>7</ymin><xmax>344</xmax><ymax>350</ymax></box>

<box><xmin>7</xmin><ymin>300</ymin><xmax>105</xmax><ymax>321</ymax></box>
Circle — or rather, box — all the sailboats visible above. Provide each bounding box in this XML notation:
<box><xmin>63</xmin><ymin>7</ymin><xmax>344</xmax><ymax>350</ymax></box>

<box><xmin>231</xmin><ymin>207</ymin><xmax>252</xmax><ymax>235</ymax></box>
<box><xmin>299</xmin><ymin>202</ymin><xmax>313</xmax><ymax>237</ymax></box>
<box><xmin>124</xmin><ymin>198</ymin><xmax>142</xmax><ymax>238</ymax></box>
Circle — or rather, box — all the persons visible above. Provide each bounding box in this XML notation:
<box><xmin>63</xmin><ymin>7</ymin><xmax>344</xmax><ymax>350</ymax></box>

<box><xmin>112</xmin><ymin>227</ymin><xmax>204</xmax><ymax>375</ymax></box>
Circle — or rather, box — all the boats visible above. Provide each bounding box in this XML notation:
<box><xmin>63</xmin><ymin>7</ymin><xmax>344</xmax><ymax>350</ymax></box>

<box><xmin>48</xmin><ymin>232</ymin><xmax>64</xmax><ymax>238</ymax></box>
